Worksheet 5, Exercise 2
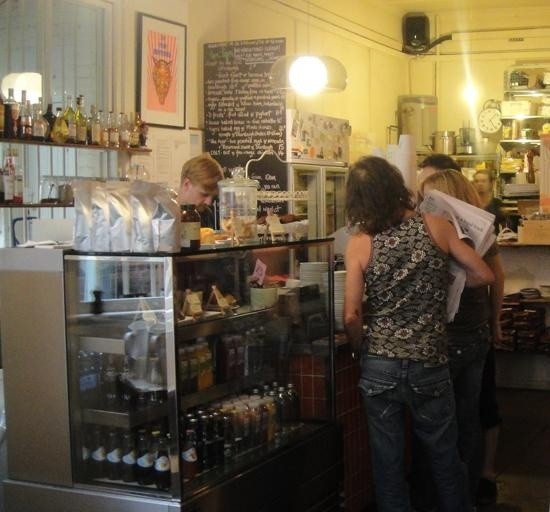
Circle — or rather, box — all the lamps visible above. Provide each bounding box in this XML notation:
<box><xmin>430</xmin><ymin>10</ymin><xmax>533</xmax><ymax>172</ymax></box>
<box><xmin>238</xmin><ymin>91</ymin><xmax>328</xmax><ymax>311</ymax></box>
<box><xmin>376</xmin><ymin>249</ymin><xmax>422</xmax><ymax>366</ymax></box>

<box><xmin>269</xmin><ymin>0</ymin><xmax>347</xmax><ymax>97</ymax></box>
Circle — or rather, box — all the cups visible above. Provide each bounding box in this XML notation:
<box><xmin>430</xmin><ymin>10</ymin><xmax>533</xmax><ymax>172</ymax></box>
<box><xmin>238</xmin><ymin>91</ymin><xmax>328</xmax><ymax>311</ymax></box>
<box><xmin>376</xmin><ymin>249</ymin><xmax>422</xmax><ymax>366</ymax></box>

<box><xmin>502</xmin><ymin>120</ymin><xmax>534</xmax><ymax>140</ymax></box>
<box><xmin>249</xmin><ymin>287</ymin><xmax>278</xmax><ymax>310</ymax></box>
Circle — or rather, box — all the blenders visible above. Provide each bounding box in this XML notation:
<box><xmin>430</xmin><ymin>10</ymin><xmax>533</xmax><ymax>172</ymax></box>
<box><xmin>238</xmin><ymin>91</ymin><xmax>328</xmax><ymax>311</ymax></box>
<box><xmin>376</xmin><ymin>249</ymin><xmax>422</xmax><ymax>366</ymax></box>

<box><xmin>399</xmin><ymin>96</ymin><xmax>437</xmax><ymax>156</ymax></box>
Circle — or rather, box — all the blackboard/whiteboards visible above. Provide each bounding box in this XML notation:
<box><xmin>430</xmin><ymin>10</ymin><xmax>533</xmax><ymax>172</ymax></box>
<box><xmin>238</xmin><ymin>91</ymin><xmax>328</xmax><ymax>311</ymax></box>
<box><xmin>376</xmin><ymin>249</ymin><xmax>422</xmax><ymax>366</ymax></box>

<box><xmin>201</xmin><ymin>37</ymin><xmax>292</xmax><ymax>218</ymax></box>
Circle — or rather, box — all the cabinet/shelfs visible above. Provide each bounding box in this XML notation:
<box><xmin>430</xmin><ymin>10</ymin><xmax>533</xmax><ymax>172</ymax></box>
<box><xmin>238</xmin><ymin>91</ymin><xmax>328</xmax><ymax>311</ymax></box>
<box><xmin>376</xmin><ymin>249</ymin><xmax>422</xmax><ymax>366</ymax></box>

<box><xmin>0</xmin><ymin>237</ymin><xmax>342</xmax><ymax>512</ymax></box>
<box><xmin>493</xmin><ymin>64</ymin><xmax>550</xmax><ymax>246</ymax></box>
<box><xmin>0</xmin><ymin>139</ymin><xmax>152</xmax><ymax>208</ymax></box>
<box><xmin>291</xmin><ymin>164</ymin><xmax>349</xmax><ymax>272</ymax></box>
<box><xmin>416</xmin><ymin>154</ymin><xmax>500</xmax><ymax>200</ymax></box>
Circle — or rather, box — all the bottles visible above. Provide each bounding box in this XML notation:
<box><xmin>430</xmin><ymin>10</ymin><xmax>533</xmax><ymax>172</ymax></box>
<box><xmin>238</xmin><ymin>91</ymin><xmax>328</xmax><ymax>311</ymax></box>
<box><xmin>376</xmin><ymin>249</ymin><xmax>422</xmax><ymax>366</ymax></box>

<box><xmin>180</xmin><ymin>202</ymin><xmax>201</xmax><ymax>252</ymax></box>
<box><xmin>0</xmin><ymin>88</ymin><xmax>148</xmax><ymax>147</ymax></box>
<box><xmin>0</xmin><ymin>148</ymin><xmax>74</xmax><ymax>205</ymax></box>
<box><xmin>220</xmin><ymin>167</ymin><xmax>261</xmax><ymax>245</ymax></box>
<box><xmin>77</xmin><ymin>327</ymin><xmax>300</xmax><ymax>490</ymax></box>
<box><xmin>434</xmin><ymin>127</ymin><xmax>475</xmax><ymax>155</ymax></box>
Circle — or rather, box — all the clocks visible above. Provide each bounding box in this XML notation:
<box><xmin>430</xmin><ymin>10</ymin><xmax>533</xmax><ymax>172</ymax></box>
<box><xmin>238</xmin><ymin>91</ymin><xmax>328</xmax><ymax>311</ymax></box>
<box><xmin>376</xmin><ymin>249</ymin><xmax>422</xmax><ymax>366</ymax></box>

<box><xmin>478</xmin><ymin>99</ymin><xmax>503</xmax><ymax>139</ymax></box>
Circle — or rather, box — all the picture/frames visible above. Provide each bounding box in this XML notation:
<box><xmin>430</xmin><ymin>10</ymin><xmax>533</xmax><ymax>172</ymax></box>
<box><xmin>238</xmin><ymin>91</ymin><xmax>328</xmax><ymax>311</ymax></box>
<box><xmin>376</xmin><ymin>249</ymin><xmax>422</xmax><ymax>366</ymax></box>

<box><xmin>135</xmin><ymin>11</ymin><xmax>187</xmax><ymax>129</ymax></box>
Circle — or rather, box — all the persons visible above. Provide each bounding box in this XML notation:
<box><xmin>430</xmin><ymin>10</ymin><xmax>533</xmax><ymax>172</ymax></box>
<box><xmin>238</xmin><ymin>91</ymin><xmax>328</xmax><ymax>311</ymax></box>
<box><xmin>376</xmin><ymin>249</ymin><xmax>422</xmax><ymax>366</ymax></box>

<box><xmin>341</xmin><ymin>156</ymin><xmax>494</xmax><ymax>511</ymax></box>
<box><xmin>473</xmin><ymin>169</ymin><xmax>506</xmax><ymax>237</ymax></box>
<box><xmin>174</xmin><ymin>154</ymin><xmax>259</xmax><ymax>304</ymax></box>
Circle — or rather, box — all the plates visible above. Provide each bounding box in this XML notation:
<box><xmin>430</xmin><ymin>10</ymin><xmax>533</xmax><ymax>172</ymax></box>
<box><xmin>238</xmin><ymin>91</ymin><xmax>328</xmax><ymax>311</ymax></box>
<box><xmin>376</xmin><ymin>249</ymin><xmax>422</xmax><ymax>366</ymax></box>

<box><xmin>299</xmin><ymin>263</ymin><xmax>346</xmax><ymax>333</ymax></box>
<box><xmin>521</xmin><ymin>285</ymin><xmax>550</xmax><ymax>300</ymax></box>
